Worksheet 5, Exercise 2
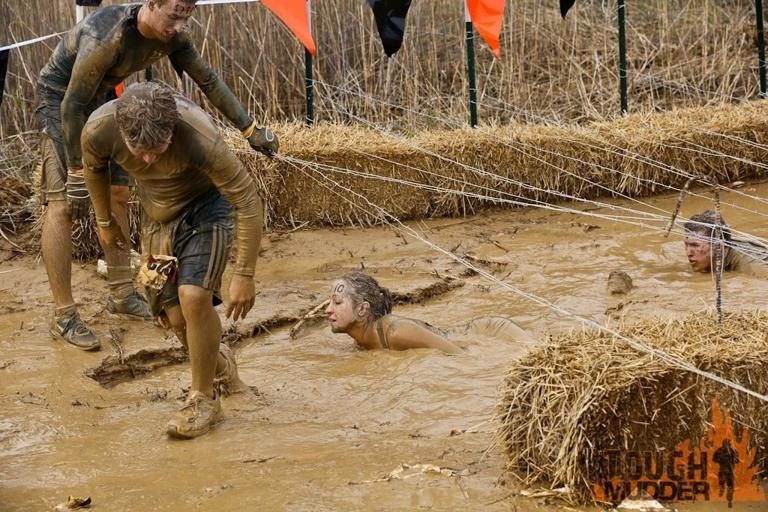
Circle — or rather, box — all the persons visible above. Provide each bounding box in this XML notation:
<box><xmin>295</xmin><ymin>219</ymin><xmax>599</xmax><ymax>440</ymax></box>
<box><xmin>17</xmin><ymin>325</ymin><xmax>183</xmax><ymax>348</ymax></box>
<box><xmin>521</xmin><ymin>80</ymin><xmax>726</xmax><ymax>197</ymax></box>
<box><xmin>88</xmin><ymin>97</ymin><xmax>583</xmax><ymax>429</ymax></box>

<box><xmin>327</xmin><ymin>270</ymin><xmax>534</xmax><ymax>357</ymax></box>
<box><xmin>683</xmin><ymin>209</ymin><xmax>768</xmax><ymax>281</ymax></box>
<box><xmin>714</xmin><ymin>439</ymin><xmax>739</xmax><ymax>507</ymax></box>
<box><xmin>80</xmin><ymin>79</ymin><xmax>264</xmax><ymax>439</ymax></box>
<box><xmin>33</xmin><ymin>0</ymin><xmax>279</xmax><ymax>351</ymax></box>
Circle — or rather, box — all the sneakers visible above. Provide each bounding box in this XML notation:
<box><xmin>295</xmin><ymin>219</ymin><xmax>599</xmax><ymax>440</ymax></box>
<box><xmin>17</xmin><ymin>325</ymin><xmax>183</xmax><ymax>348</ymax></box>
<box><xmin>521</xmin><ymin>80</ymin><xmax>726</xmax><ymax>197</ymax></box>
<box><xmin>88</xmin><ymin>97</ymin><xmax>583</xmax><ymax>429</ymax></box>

<box><xmin>48</xmin><ymin>313</ymin><xmax>101</xmax><ymax>351</ymax></box>
<box><xmin>105</xmin><ymin>294</ymin><xmax>160</xmax><ymax>318</ymax></box>
<box><xmin>608</xmin><ymin>269</ymin><xmax>632</xmax><ymax>295</ymax></box>
<box><xmin>213</xmin><ymin>343</ymin><xmax>240</xmax><ymax>403</ymax></box>
<box><xmin>167</xmin><ymin>387</ymin><xmax>224</xmax><ymax>440</ymax></box>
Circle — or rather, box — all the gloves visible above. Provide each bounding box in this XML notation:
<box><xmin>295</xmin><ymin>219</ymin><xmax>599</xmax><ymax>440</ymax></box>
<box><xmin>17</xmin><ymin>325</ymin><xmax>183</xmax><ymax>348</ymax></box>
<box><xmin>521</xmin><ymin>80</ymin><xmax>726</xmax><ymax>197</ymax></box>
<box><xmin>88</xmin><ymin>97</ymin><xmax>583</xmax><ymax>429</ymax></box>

<box><xmin>247</xmin><ymin>122</ymin><xmax>279</xmax><ymax>158</ymax></box>
<box><xmin>65</xmin><ymin>169</ymin><xmax>92</xmax><ymax>222</ymax></box>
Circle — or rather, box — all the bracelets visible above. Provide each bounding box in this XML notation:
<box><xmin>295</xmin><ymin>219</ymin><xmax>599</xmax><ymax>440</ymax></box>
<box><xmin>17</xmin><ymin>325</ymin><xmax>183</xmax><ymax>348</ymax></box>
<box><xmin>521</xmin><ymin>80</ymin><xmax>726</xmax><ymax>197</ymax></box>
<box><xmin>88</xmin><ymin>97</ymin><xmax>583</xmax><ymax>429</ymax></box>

<box><xmin>243</xmin><ymin>121</ymin><xmax>257</xmax><ymax>138</ymax></box>
<box><xmin>98</xmin><ymin>221</ymin><xmax>116</xmax><ymax>229</ymax></box>
